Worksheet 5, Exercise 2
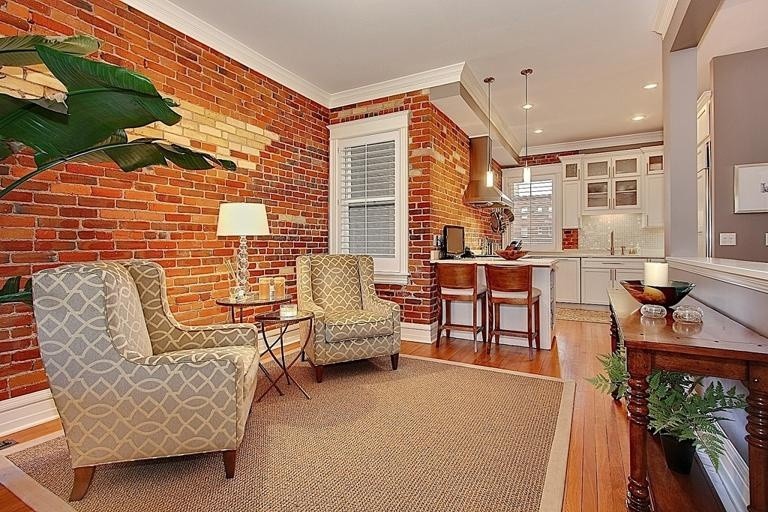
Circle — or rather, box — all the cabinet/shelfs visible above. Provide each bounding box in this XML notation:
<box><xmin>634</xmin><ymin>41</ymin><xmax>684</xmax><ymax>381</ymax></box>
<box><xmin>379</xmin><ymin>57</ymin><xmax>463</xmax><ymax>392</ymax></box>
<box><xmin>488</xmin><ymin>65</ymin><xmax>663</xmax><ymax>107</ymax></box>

<box><xmin>554</xmin><ymin>258</ymin><xmax>579</xmax><ymax>302</ymax></box>
<box><xmin>581</xmin><ymin>148</ymin><xmax>640</xmax><ymax>177</ymax></box>
<box><xmin>581</xmin><ymin>257</ymin><xmax>645</xmax><ymax>306</ymax></box>
<box><xmin>697</xmin><ymin>167</ymin><xmax>709</xmax><ymax>257</ymax></box>
<box><xmin>582</xmin><ymin>176</ymin><xmax>641</xmax><ymax>216</ymax></box>
<box><xmin>697</xmin><ymin>90</ymin><xmax>713</xmax><ymax>145</ymax></box>
<box><xmin>557</xmin><ymin>154</ymin><xmax>581</xmax><ymax>233</ymax></box>
<box><xmin>641</xmin><ymin>144</ymin><xmax>664</xmax><ymax>228</ymax></box>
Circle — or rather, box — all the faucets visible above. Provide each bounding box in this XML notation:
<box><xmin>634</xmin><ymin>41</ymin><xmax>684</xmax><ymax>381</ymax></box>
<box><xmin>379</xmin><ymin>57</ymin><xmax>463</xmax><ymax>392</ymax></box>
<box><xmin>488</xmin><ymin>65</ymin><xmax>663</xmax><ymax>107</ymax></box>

<box><xmin>610</xmin><ymin>230</ymin><xmax>615</xmax><ymax>256</ymax></box>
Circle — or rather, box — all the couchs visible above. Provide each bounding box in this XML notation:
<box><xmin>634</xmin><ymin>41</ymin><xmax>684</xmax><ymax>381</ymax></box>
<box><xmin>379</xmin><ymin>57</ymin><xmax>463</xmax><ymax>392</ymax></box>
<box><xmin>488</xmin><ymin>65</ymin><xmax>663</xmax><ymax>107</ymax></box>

<box><xmin>291</xmin><ymin>252</ymin><xmax>403</xmax><ymax>382</ymax></box>
<box><xmin>31</xmin><ymin>259</ymin><xmax>260</xmax><ymax>503</ymax></box>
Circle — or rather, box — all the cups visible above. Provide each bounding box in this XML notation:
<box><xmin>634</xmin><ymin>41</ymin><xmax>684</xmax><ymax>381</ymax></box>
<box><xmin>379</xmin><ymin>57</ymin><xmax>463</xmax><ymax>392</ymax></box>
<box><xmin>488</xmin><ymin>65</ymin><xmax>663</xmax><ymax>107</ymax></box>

<box><xmin>274</xmin><ymin>277</ymin><xmax>285</xmax><ymax>299</ymax></box>
<box><xmin>259</xmin><ymin>277</ymin><xmax>273</xmax><ymax>298</ymax></box>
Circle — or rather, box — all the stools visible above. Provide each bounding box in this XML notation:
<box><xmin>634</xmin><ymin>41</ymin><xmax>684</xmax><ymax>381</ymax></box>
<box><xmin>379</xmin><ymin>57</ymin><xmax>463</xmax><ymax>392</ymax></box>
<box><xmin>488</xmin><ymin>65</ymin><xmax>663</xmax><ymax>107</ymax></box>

<box><xmin>255</xmin><ymin>311</ymin><xmax>311</xmax><ymax>402</ymax></box>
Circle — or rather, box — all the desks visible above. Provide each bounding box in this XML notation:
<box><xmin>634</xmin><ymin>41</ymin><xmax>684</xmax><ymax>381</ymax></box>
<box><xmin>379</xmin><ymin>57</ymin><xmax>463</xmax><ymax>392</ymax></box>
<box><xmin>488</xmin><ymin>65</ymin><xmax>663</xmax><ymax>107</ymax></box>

<box><xmin>606</xmin><ymin>288</ymin><xmax>767</xmax><ymax>512</ymax></box>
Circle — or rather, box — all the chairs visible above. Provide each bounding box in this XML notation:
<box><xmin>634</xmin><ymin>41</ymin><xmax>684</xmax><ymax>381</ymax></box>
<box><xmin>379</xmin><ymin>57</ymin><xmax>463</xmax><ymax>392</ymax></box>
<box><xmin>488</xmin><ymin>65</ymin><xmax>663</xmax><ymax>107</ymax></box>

<box><xmin>435</xmin><ymin>261</ymin><xmax>487</xmax><ymax>353</ymax></box>
<box><xmin>482</xmin><ymin>261</ymin><xmax>541</xmax><ymax>361</ymax></box>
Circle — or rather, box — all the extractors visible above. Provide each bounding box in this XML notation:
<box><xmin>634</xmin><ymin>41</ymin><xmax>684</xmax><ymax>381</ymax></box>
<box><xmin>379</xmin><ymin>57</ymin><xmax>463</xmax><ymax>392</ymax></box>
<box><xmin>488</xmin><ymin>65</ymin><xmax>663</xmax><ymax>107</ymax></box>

<box><xmin>462</xmin><ymin>137</ymin><xmax>514</xmax><ymax>208</ymax></box>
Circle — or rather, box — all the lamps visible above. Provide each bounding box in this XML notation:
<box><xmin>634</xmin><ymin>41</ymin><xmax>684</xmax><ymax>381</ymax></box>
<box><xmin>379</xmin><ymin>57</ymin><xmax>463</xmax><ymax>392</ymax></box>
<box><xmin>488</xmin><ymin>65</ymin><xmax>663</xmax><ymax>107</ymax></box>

<box><xmin>216</xmin><ymin>203</ymin><xmax>268</xmax><ymax>300</ymax></box>
<box><xmin>483</xmin><ymin>76</ymin><xmax>495</xmax><ymax>187</ymax></box>
<box><xmin>520</xmin><ymin>68</ymin><xmax>532</xmax><ymax>182</ymax></box>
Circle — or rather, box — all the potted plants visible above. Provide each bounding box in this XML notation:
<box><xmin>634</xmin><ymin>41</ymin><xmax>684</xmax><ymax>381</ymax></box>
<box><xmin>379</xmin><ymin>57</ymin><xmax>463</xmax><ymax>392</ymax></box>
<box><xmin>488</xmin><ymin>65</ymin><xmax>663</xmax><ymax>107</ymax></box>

<box><xmin>589</xmin><ymin>346</ymin><xmax>745</xmax><ymax>476</ymax></box>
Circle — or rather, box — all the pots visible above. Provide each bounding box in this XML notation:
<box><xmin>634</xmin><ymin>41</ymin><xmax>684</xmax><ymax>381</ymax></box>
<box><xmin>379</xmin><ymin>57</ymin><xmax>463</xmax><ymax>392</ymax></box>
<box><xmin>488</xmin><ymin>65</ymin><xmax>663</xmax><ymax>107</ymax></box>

<box><xmin>490</xmin><ymin>208</ymin><xmax>514</xmax><ymax>234</ymax></box>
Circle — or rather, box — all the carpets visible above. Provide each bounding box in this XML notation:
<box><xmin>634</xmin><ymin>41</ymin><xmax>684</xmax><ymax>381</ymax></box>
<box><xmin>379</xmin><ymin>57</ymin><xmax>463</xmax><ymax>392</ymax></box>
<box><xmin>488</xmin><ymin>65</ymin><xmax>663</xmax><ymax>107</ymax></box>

<box><xmin>555</xmin><ymin>305</ymin><xmax>611</xmax><ymax>324</ymax></box>
<box><xmin>0</xmin><ymin>345</ymin><xmax>577</xmax><ymax>512</ymax></box>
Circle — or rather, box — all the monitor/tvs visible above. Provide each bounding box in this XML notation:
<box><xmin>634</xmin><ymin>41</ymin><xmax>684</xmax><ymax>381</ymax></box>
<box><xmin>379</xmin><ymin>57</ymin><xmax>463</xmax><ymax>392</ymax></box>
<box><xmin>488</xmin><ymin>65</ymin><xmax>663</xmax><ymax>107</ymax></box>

<box><xmin>444</xmin><ymin>225</ymin><xmax>464</xmax><ymax>259</ymax></box>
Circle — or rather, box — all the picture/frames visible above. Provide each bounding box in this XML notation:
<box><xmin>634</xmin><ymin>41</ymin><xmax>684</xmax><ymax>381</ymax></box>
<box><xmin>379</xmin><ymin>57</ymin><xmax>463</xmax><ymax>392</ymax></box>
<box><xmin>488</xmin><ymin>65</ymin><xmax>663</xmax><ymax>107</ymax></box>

<box><xmin>733</xmin><ymin>160</ymin><xmax>767</xmax><ymax>215</ymax></box>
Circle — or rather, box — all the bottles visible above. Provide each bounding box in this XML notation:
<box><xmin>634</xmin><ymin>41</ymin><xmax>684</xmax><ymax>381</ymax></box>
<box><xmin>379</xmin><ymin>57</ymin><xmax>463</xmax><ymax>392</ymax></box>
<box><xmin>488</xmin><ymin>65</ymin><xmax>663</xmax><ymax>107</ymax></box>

<box><xmin>478</xmin><ymin>236</ymin><xmax>499</xmax><ymax>255</ymax></box>
<box><xmin>269</xmin><ymin>279</ymin><xmax>275</xmax><ymax>298</ymax></box>
<box><xmin>628</xmin><ymin>242</ymin><xmax>640</xmax><ymax>256</ymax></box>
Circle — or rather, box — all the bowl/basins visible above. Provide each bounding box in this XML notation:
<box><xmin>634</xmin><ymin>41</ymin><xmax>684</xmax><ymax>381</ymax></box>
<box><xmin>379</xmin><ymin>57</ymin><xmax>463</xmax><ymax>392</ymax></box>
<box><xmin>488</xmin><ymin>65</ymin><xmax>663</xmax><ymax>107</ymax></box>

<box><xmin>280</xmin><ymin>304</ymin><xmax>298</xmax><ymax>317</ymax></box>
<box><xmin>620</xmin><ymin>279</ymin><xmax>695</xmax><ymax>307</ymax></box>
<box><xmin>496</xmin><ymin>250</ymin><xmax>530</xmax><ymax>260</ymax></box>
